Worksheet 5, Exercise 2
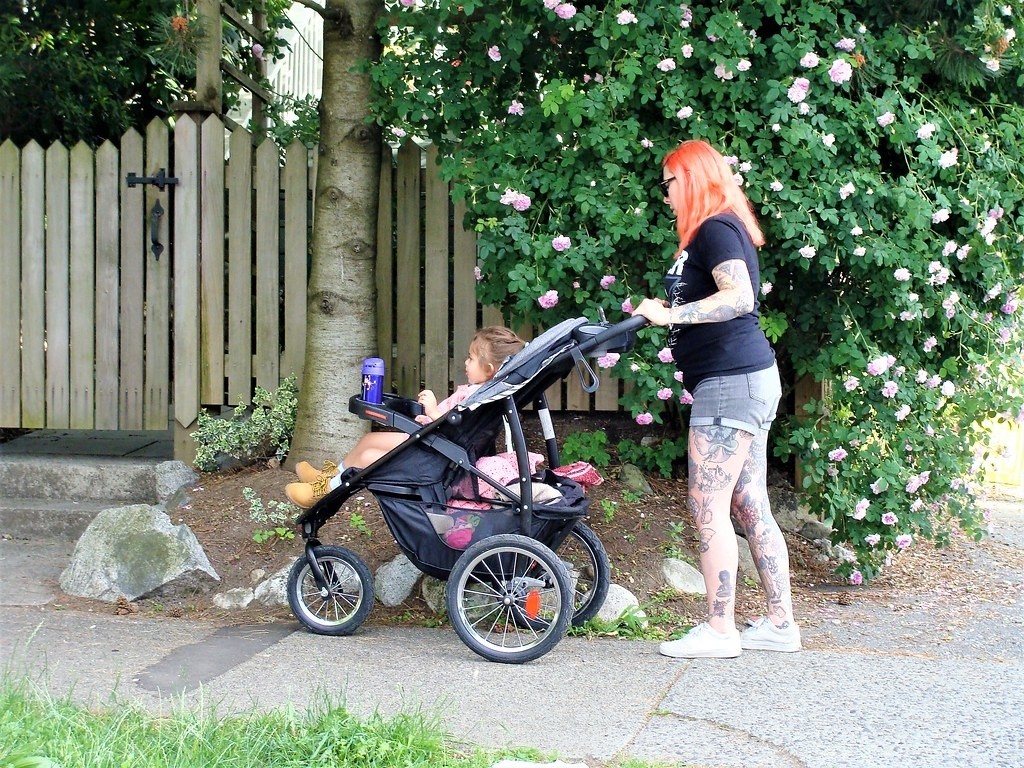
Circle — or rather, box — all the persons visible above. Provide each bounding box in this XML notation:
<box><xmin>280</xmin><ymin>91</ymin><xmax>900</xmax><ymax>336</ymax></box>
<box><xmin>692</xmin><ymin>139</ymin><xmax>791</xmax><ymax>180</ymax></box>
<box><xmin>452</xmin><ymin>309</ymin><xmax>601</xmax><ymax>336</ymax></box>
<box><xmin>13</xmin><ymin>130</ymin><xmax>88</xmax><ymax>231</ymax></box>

<box><xmin>631</xmin><ymin>139</ymin><xmax>801</xmax><ymax>657</ymax></box>
<box><xmin>284</xmin><ymin>325</ymin><xmax>529</xmax><ymax>510</ymax></box>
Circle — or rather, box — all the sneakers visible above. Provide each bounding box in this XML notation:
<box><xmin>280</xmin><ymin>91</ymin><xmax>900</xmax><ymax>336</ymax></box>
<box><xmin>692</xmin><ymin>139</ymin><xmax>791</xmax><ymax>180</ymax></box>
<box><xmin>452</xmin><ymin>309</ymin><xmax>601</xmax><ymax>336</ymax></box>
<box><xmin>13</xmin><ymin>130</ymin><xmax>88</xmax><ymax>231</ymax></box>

<box><xmin>738</xmin><ymin>616</ymin><xmax>801</xmax><ymax>653</ymax></box>
<box><xmin>659</xmin><ymin>622</ymin><xmax>741</xmax><ymax>658</ymax></box>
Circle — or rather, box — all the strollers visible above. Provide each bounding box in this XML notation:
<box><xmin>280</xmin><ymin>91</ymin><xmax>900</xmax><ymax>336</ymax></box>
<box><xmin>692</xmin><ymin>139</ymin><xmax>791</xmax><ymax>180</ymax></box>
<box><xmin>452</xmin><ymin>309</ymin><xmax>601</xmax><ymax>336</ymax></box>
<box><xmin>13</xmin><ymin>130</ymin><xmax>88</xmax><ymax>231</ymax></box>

<box><xmin>286</xmin><ymin>312</ymin><xmax>655</xmax><ymax>664</ymax></box>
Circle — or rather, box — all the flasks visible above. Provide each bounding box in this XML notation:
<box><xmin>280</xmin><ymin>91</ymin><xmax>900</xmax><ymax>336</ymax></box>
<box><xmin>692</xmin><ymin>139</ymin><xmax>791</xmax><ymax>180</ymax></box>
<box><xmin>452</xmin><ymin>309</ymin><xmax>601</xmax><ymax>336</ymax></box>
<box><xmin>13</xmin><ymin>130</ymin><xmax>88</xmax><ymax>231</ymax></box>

<box><xmin>361</xmin><ymin>358</ymin><xmax>385</xmax><ymax>402</ymax></box>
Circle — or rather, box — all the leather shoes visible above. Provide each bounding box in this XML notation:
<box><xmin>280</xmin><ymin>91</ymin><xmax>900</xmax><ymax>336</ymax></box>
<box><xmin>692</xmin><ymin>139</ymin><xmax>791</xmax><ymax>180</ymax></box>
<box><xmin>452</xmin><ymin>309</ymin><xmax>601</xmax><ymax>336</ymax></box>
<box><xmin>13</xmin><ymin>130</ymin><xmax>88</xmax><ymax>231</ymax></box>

<box><xmin>295</xmin><ymin>461</ymin><xmax>332</xmax><ymax>483</ymax></box>
<box><xmin>284</xmin><ymin>480</ymin><xmax>331</xmax><ymax>510</ymax></box>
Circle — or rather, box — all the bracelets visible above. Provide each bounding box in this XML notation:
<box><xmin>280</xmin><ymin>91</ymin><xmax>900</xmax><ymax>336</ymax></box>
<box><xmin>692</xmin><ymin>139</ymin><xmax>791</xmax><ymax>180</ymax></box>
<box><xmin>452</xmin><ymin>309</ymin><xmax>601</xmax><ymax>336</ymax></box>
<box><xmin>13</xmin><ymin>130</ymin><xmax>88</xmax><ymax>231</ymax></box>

<box><xmin>668</xmin><ymin>307</ymin><xmax>674</xmax><ymax>331</ymax></box>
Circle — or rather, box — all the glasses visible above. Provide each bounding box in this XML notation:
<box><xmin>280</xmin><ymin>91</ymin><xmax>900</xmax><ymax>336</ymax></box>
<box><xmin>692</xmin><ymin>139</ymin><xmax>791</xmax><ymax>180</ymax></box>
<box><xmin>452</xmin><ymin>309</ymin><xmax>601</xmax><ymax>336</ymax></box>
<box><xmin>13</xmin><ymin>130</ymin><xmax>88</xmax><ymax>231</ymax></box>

<box><xmin>657</xmin><ymin>177</ymin><xmax>675</xmax><ymax>198</ymax></box>
